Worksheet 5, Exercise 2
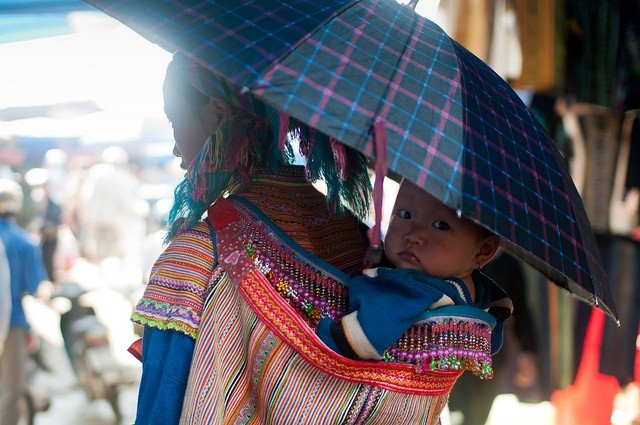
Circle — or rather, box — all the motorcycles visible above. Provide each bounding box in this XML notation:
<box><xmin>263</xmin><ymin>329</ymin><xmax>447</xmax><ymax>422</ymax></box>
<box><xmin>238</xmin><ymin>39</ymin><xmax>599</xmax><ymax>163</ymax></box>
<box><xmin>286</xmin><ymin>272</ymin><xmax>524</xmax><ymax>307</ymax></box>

<box><xmin>50</xmin><ymin>288</ymin><xmax>134</xmax><ymax>420</ymax></box>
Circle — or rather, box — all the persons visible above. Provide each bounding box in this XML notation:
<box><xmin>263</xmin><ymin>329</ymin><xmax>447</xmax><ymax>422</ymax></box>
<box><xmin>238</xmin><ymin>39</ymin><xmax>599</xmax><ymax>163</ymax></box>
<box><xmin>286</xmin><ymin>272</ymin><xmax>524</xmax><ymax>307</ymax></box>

<box><xmin>316</xmin><ymin>175</ymin><xmax>514</xmax><ymax>381</ymax></box>
<box><xmin>447</xmin><ymin>249</ymin><xmax>540</xmax><ymax>425</ymax></box>
<box><xmin>25</xmin><ymin>148</ymin><xmax>175</xmax><ymax>288</ymax></box>
<box><xmin>0</xmin><ymin>177</ymin><xmax>56</xmax><ymax>425</ymax></box>
<box><xmin>126</xmin><ymin>52</ymin><xmax>395</xmax><ymax>424</ymax></box>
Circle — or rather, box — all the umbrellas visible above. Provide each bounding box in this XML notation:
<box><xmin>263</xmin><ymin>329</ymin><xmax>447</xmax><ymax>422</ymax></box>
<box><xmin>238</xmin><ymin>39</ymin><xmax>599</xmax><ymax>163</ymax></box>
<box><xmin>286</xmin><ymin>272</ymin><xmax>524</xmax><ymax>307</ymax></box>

<box><xmin>83</xmin><ymin>0</ymin><xmax>623</xmax><ymax>330</ymax></box>
